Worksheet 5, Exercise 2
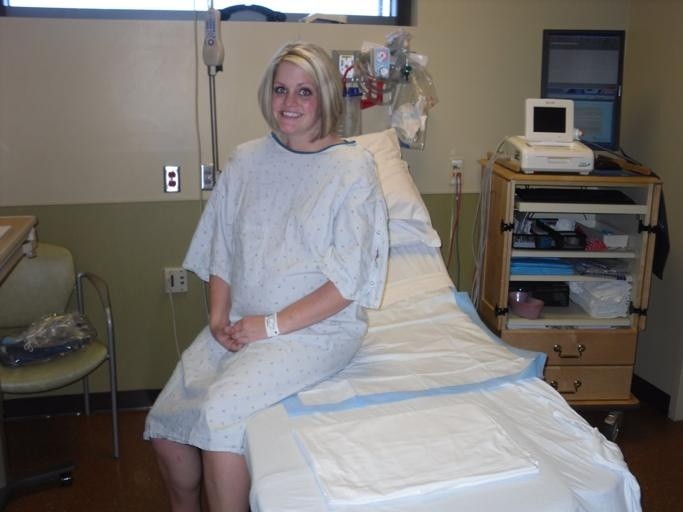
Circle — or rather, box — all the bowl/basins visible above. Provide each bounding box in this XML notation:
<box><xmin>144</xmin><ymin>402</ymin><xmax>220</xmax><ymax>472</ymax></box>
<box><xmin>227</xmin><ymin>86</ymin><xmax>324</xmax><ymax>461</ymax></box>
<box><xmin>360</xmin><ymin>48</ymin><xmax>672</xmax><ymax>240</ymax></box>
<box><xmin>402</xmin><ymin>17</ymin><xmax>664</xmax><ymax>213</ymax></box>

<box><xmin>508</xmin><ymin>292</ymin><xmax>543</xmax><ymax>319</ymax></box>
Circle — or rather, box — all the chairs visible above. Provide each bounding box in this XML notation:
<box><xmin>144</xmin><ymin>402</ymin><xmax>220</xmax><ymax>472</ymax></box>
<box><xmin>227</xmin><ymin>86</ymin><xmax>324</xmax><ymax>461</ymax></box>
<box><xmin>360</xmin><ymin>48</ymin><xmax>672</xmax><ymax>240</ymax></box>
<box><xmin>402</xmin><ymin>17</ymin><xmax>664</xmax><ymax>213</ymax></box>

<box><xmin>0</xmin><ymin>243</ymin><xmax>122</xmax><ymax>459</ymax></box>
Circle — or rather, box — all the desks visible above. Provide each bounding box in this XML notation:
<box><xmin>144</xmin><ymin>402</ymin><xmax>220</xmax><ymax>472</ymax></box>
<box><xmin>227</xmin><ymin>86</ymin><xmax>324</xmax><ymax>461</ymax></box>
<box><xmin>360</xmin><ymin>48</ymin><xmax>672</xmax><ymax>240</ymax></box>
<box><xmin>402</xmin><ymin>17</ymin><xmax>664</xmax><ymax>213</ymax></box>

<box><xmin>0</xmin><ymin>214</ymin><xmax>83</xmax><ymax>511</ymax></box>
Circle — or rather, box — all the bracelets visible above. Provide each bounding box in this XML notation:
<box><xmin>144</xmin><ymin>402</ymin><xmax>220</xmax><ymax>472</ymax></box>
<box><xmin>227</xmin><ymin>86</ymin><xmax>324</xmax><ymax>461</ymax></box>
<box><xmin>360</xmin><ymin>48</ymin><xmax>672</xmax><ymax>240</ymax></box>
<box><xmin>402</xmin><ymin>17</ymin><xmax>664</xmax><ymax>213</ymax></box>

<box><xmin>261</xmin><ymin>311</ymin><xmax>280</xmax><ymax>338</ymax></box>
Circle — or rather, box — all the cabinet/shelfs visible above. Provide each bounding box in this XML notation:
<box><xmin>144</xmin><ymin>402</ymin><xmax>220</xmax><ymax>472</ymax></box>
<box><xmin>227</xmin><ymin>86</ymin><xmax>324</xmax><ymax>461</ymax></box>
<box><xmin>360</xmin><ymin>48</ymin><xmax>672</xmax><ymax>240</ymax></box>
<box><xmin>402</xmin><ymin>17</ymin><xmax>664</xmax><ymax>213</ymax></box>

<box><xmin>467</xmin><ymin>154</ymin><xmax>664</xmax><ymax>445</ymax></box>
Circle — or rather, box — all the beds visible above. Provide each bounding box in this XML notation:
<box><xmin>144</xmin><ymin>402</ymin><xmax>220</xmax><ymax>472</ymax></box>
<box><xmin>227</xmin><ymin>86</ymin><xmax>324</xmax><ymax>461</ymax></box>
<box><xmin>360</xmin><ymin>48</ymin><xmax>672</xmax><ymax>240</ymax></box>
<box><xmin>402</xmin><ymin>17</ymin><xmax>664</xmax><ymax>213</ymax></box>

<box><xmin>237</xmin><ymin>246</ymin><xmax>641</xmax><ymax>511</ymax></box>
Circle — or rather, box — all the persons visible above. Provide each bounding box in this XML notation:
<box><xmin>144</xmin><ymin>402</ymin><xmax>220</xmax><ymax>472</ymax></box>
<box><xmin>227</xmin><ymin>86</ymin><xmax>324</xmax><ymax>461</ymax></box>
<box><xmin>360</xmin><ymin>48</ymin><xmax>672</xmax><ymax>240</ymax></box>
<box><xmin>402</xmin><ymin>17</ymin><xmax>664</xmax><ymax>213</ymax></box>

<box><xmin>143</xmin><ymin>39</ymin><xmax>390</xmax><ymax>512</ymax></box>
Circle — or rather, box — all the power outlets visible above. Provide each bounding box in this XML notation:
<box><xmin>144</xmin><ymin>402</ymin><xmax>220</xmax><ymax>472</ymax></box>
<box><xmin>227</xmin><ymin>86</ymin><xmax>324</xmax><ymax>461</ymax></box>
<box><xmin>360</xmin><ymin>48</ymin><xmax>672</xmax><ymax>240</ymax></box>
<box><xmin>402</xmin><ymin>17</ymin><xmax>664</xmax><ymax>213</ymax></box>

<box><xmin>448</xmin><ymin>158</ymin><xmax>466</xmax><ymax>187</ymax></box>
<box><xmin>162</xmin><ymin>266</ymin><xmax>189</xmax><ymax>295</ymax></box>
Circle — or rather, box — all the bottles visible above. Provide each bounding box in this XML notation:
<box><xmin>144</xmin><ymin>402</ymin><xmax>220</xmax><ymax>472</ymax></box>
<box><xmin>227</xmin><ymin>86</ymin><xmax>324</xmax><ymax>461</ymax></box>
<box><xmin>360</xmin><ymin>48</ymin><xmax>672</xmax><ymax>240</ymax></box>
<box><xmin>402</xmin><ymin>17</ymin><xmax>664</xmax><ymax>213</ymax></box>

<box><xmin>341</xmin><ymin>88</ymin><xmax>363</xmax><ymax>137</ymax></box>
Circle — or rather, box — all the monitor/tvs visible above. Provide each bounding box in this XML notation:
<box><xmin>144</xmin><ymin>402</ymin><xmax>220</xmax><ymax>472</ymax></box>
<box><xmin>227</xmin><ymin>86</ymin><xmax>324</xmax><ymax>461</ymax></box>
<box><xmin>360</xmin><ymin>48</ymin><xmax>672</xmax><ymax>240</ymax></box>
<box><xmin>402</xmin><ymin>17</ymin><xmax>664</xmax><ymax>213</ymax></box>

<box><xmin>525</xmin><ymin>98</ymin><xmax>574</xmax><ymax>141</ymax></box>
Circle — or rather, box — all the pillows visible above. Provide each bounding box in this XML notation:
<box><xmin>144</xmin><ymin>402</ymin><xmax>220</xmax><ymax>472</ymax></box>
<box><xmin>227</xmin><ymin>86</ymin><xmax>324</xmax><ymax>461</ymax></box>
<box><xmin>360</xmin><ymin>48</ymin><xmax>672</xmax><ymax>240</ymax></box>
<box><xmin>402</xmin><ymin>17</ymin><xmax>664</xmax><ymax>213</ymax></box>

<box><xmin>234</xmin><ymin>127</ymin><xmax>442</xmax><ymax>249</ymax></box>
